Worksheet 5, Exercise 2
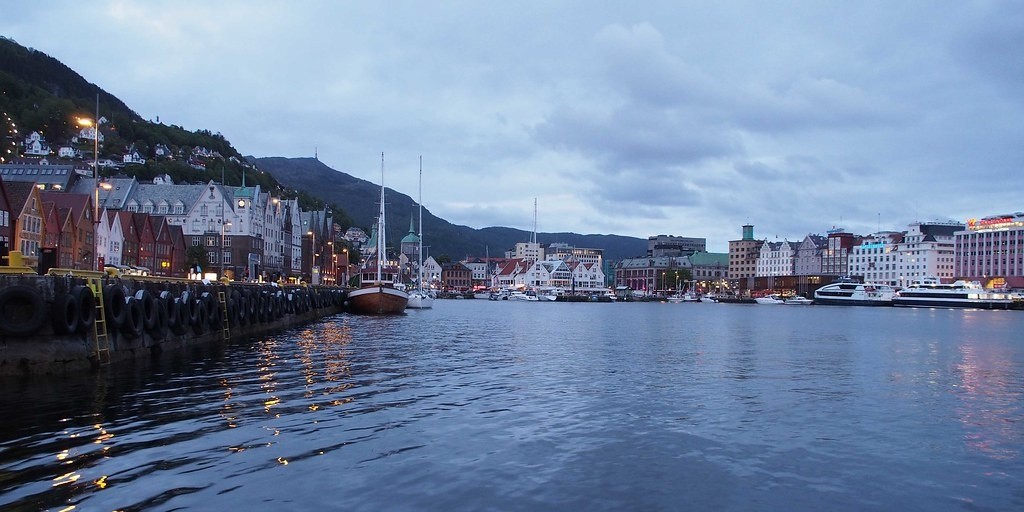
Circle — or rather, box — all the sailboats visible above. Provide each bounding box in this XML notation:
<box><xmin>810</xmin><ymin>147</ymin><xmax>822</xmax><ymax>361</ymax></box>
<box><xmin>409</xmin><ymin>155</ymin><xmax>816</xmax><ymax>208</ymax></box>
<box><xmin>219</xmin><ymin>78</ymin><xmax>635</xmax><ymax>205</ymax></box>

<box><xmin>403</xmin><ymin>155</ymin><xmax>434</xmax><ymax>310</ymax></box>
<box><xmin>349</xmin><ymin>151</ymin><xmax>411</xmax><ymax>316</ymax></box>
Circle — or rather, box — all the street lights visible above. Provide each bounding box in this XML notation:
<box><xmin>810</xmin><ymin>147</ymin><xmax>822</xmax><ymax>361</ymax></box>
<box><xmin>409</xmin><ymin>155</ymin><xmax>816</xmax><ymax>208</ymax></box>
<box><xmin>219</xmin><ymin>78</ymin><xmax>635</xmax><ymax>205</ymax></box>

<box><xmin>343</xmin><ymin>248</ymin><xmax>349</xmax><ymax>286</ymax></box>
<box><xmin>662</xmin><ymin>272</ymin><xmax>665</xmax><ymax>290</ymax></box>
<box><xmin>333</xmin><ymin>255</ymin><xmax>338</xmax><ymax>286</ymax></box>
<box><xmin>306</xmin><ymin>230</ymin><xmax>315</xmax><ymax>267</ymax></box>
<box><xmin>676</xmin><ymin>276</ymin><xmax>679</xmax><ymax>289</ymax></box>
<box><xmin>327</xmin><ymin>241</ymin><xmax>334</xmax><ymax>287</ymax></box>
<box><xmin>220</xmin><ymin>221</ymin><xmax>233</xmax><ymax>278</ymax></box>
<box><xmin>77</xmin><ymin>116</ymin><xmax>112</xmax><ymax>273</ymax></box>
<box><xmin>273</xmin><ymin>195</ymin><xmax>281</xmax><ymax>276</ymax></box>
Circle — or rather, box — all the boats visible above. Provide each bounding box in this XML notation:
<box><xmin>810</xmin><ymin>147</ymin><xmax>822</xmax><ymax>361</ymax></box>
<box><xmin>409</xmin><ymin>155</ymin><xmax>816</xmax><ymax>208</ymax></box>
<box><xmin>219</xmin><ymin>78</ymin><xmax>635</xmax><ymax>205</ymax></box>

<box><xmin>486</xmin><ymin>195</ymin><xmax>816</xmax><ymax>305</ymax></box>
<box><xmin>892</xmin><ymin>282</ymin><xmax>1024</xmax><ymax>307</ymax></box>
<box><xmin>813</xmin><ymin>281</ymin><xmax>901</xmax><ymax>306</ymax></box>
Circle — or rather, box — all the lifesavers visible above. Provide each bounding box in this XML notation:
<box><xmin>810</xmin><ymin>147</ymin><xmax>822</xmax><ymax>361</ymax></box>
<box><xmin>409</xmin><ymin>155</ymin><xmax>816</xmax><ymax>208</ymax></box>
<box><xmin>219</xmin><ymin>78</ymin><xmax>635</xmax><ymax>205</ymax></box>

<box><xmin>53</xmin><ymin>294</ymin><xmax>78</xmax><ymax>336</ymax></box>
<box><xmin>102</xmin><ymin>283</ymin><xmax>127</xmax><ymax>329</ymax></box>
<box><xmin>0</xmin><ymin>285</ymin><xmax>47</xmax><ymax>338</ymax></box>
<box><xmin>124</xmin><ymin>289</ymin><xmax>346</xmax><ymax>341</ymax></box>
<box><xmin>71</xmin><ymin>284</ymin><xmax>97</xmax><ymax>335</ymax></box>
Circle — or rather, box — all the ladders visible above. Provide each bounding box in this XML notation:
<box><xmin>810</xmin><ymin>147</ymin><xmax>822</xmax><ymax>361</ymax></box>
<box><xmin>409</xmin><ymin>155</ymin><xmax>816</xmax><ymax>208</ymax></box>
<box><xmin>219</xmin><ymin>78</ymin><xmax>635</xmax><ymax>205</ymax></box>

<box><xmin>217</xmin><ymin>290</ymin><xmax>232</xmax><ymax>341</ymax></box>
<box><xmin>86</xmin><ymin>275</ymin><xmax>112</xmax><ymax>365</ymax></box>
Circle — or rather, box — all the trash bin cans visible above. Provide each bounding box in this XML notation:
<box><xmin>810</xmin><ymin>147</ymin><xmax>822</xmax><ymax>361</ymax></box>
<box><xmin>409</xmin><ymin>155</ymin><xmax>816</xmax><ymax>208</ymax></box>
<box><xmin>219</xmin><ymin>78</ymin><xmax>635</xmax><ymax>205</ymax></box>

<box><xmin>37</xmin><ymin>244</ymin><xmax>58</xmax><ymax>276</ymax></box>
